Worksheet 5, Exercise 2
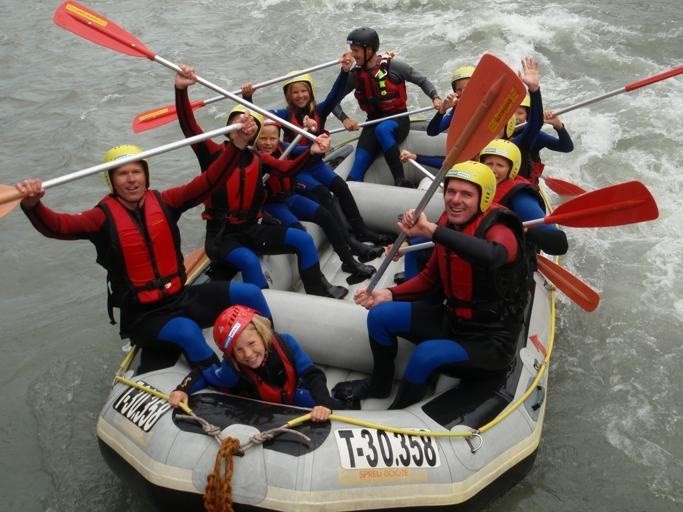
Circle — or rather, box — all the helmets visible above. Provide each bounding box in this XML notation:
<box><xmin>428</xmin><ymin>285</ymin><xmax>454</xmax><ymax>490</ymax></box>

<box><xmin>257</xmin><ymin>108</ymin><xmax>282</xmax><ymax>129</ymax></box>
<box><xmin>104</xmin><ymin>144</ymin><xmax>152</xmax><ymax>193</ymax></box>
<box><xmin>281</xmin><ymin>68</ymin><xmax>316</xmax><ymax>105</ymax></box>
<box><xmin>477</xmin><ymin>138</ymin><xmax>523</xmax><ymax>179</ymax></box>
<box><xmin>450</xmin><ymin>64</ymin><xmax>476</xmax><ymax>83</ymax></box>
<box><xmin>226</xmin><ymin>104</ymin><xmax>264</xmax><ymax>146</ymax></box>
<box><xmin>345</xmin><ymin>26</ymin><xmax>380</xmax><ymax>53</ymax></box>
<box><xmin>211</xmin><ymin>304</ymin><xmax>262</xmax><ymax>359</ymax></box>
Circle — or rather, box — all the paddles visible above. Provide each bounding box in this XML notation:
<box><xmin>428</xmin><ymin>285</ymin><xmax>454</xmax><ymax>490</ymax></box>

<box><xmin>405</xmin><ymin>157</ymin><xmax>600</xmax><ymax>312</ymax></box>
<box><xmin>132</xmin><ymin>58</ymin><xmax>345</xmax><ymax>132</ymax></box>
<box><xmin>0</xmin><ymin>123</ymin><xmax>248</xmax><ymax>217</ymax></box>
<box><xmin>511</xmin><ymin>66</ymin><xmax>683</xmax><ymax>131</ymax></box>
<box><xmin>384</xmin><ymin>181</ymin><xmax>658</xmax><ymax>261</ymax></box>
<box><xmin>54</xmin><ymin>0</ymin><xmax>323</xmax><ymax>143</ymax></box>
<box><xmin>367</xmin><ymin>54</ymin><xmax>526</xmax><ymax>295</ymax></box>
<box><xmin>539</xmin><ymin>173</ymin><xmax>586</xmax><ymax>196</ymax></box>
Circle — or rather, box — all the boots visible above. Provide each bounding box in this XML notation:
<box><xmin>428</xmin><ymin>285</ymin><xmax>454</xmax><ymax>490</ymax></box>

<box><xmin>386</xmin><ymin>374</ymin><xmax>427</xmax><ymax>409</ymax></box>
<box><xmin>384</xmin><ymin>144</ymin><xmax>411</xmax><ymax>187</ymax></box>
<box><xmin>298</xmin><ymin>263</ymin><xmax>347</xmax><ymax>298</ymax></box>
<box><xmin>314</xmin><ymin>175</ymin><xmax>397</xmax><ymax>277</ymax></box>
<box><xmin>332</xmin><ymin>339</ymin><xmax>398</xmax><ymax>400</ymax></box>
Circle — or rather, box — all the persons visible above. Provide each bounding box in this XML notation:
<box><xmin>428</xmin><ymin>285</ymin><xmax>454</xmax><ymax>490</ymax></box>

<box><xmin>240</xmin><ymin>52</ymin><xmax>399</xmax><ymax>262</ymax></box>
<box><xmin>170</xmin><ymin>305</ymin><xmax>333</xmax><ymax>424</ymax></box>
<box><xmin>394</xmin><ymin>140</ymin><xmax>568</xmax><ymax>285</ymax></box>
<box><xmin>16</xmin><ymin>109</ymin><xmax>274</xmax><ymax>382</ymax></box>
<box><xmin>334</xmin><ymin>161</ymin><xmax>532</xmax><ymax>408</ymax></box>
<box><xmin>249</xmin><ymin>116</ymin><xmax>378</xmax><ymax>302</ymax></box>
<box><xmin>332</xmin><ymin>27</ymin><xmax>441</xmax><ymax>191</ymax></box>
<box><xmin>174</xmin><ymin>64</ymin><xmax>347</xmax><ymax>301</ymax></box>
<box><xmin>400</xmin><ymin>57</ymin><xmax>575</xmax><ymax>257</ymax></box>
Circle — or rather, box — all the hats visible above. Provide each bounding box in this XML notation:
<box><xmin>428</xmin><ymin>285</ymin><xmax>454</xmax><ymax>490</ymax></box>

<box><xmin>443</xmin><ymin>160</ymin><xmax>497</xmax><ymax>214</ymax></box>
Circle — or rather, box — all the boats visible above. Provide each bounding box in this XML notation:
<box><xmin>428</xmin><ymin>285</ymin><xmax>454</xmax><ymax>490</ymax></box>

<box><xmin>93</xmin><ymin>118</ymin><xmax>561</xmax><ymax>510</ymax></box>
